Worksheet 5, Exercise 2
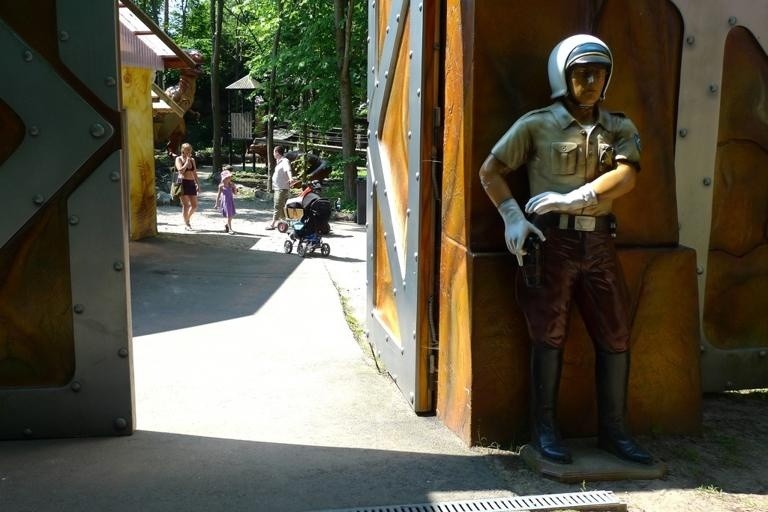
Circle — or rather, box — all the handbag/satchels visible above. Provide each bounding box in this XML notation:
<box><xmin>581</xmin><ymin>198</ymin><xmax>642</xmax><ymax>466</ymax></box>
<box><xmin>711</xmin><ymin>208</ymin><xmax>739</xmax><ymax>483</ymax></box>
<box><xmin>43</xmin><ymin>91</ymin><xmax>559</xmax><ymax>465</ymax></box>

<box><xmin>171</xmin><ymin>181</ymin><xmax>184</xmax><ymax>201</ymax></box>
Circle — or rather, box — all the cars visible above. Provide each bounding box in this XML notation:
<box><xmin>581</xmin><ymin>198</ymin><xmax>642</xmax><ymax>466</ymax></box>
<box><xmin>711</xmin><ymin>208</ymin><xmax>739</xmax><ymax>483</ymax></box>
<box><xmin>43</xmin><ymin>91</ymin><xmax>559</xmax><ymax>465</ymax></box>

<box><xmin>244</xmin><ymin>148</ymin><xmax>260</xmax><ymax>164</ymax></box>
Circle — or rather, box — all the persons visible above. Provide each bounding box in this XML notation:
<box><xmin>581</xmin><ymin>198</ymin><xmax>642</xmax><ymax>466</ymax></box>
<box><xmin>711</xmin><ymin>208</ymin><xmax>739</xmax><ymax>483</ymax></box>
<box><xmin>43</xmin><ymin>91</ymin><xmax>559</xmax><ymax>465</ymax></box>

<box><xmin>264</xmin><ymin>145</ymin><xmax>294</xmax><ymax>230</ymax></box>
<box><xmin>214</xmin><ymin>169</ymin><xmax>238</xmax><ymax>235</ymax></box>
<box><xmin>174</xmin><ymin>142</ymin><xmax>200</xmax><ymax>232</ymax></box>
<box><xmin>477</xmin><ymin>32</ymin><xmax>656</xmax><ymax>465</ymax></box>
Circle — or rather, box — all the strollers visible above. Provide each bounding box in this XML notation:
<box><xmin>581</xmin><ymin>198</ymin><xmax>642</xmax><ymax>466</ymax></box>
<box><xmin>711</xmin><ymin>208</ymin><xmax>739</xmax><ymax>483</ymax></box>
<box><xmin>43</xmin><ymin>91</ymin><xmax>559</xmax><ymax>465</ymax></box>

<box><xmin>276</xmin><ymin>194</ymin><xmax>332</xmax><ymax>237</ymax></box>
<box><xmin>281</xmin><ymin>189</ymin><xmax>334</xmax><ymax>260</ymax></box>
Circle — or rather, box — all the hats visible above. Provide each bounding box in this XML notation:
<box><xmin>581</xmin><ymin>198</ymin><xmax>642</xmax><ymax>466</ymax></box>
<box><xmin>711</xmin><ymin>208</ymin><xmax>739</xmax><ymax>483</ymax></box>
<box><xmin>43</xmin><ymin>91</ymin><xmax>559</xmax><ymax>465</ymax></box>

<box><xmin>220</xmin><ymin>170</ymin><xmax>232</xmax><ymax>183</ymax></box>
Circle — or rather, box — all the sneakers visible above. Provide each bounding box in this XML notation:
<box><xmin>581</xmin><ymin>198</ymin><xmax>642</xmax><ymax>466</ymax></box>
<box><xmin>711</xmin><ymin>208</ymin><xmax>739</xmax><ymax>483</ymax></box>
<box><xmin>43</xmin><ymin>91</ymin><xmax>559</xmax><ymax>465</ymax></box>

<box><xmin>185</xmin><ymin>224</ymin><xmax>193</xmax><ymax>231</ymax></box>
<box><xmin>225</xmin><ymin>224</ymin><xmax>238</xmax><ymax>235</ymax></box>
<box><xmin>265</xmin><ymin>225</ymin><xmax>274</xmax><ymax>230</ymax></box>
